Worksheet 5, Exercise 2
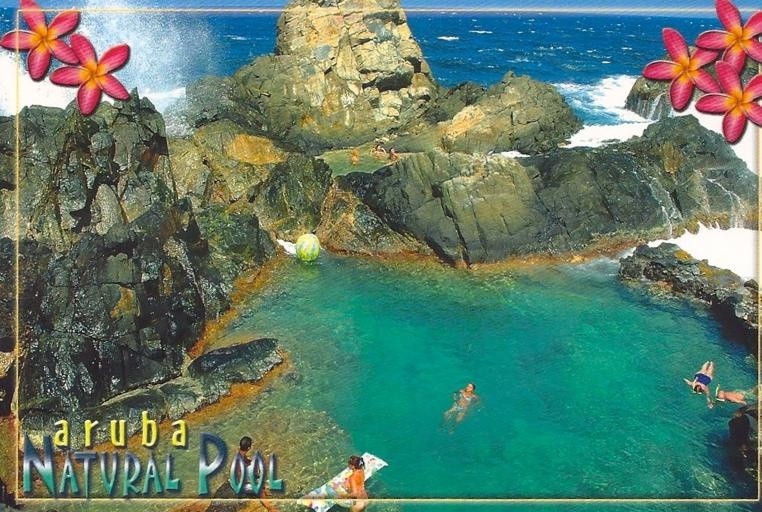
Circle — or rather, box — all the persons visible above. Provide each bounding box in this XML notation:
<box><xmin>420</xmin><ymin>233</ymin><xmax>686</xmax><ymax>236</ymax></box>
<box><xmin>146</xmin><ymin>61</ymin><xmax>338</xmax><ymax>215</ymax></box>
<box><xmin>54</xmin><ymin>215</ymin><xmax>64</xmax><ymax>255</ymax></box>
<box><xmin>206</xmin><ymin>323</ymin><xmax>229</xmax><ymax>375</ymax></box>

<box><xmin>333</xmin><ymin>456</ymin><xmax>370</xmax><ymax>511</ymax></box>
<box><xmin>442</xmin><ymin>381</ymin><xmax>479</xmax><ymax>426</ymax></box>
<box><xmin>713</xmin><ymin>382</ymin><xmax>759</xmax><ymax>406</ymax></box>
<box><xmin>681</xmin><ymin>358</ymin><xmax>714</xmax><ymax>412</ymax></box>
<box><xmin>236</xmin><ymin>435</ymin><xmax>277</xmax><ymax>512</ymax></box>
